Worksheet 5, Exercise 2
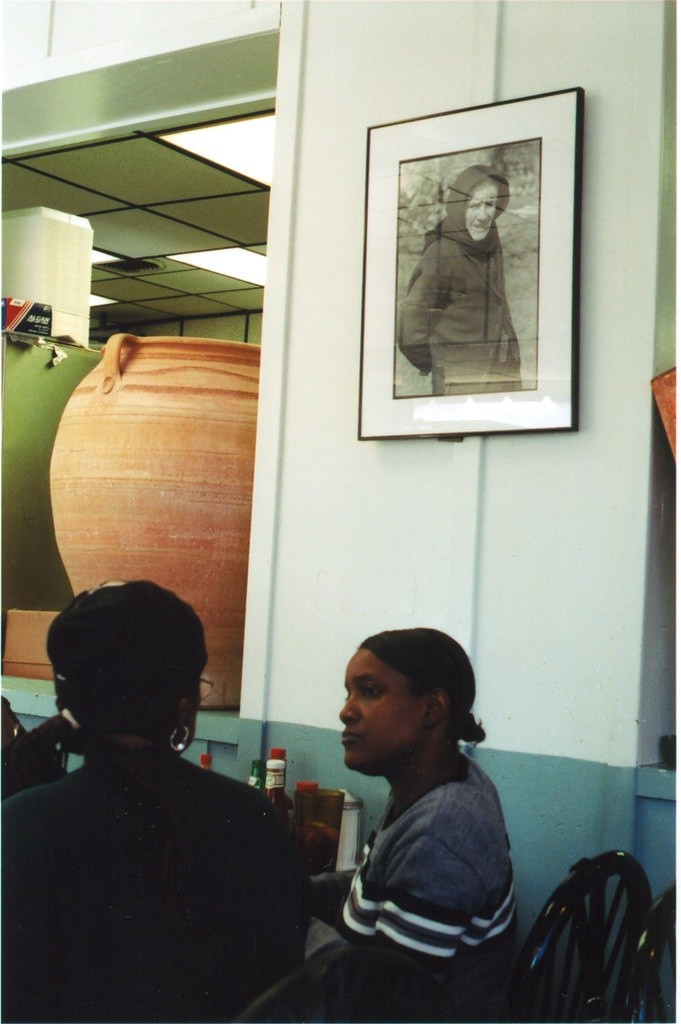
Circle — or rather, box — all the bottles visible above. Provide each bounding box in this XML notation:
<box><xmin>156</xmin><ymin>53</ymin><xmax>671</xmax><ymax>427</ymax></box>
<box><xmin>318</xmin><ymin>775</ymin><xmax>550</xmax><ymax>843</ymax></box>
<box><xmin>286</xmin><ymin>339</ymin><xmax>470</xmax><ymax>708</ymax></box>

<box><xmin>249</xmin><ymin>749</ymin><xmax>318</xmax><ymax>830</ymax></box>
<box><xmin>47</xmin><ymin>332</ymin><xmax>258</xmax><ymax>707</ymax></box>
<box><xmin>199</xmin><ymin>754</ymin><xmax>212</xmax><ymax>771</ymax></box>
<box><xmin>337</xmin><ymin>789</ymin><xmax>363</xmax><ymax>872</ymax></box>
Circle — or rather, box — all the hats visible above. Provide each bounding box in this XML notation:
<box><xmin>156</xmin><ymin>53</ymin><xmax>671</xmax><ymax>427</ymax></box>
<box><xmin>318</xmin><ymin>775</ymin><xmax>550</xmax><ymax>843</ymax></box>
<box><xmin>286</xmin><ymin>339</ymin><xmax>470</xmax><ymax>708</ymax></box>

<box><xmin>47</xmin><ymin>581</ymin><xmax>208</xmax><ymax>714</ymax></box>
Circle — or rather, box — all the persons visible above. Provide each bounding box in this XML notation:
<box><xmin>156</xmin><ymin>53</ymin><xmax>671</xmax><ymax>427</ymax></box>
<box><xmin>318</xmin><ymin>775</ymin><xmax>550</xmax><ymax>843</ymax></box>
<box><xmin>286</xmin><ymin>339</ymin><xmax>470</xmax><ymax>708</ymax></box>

<box><xmin>399</xmin><ymin>164</ymin><xmax>522</xmax><ymax>394</ymax></box>
<box><xmin>279</xmin><ymin>629</ymin><xmax>518</xmax><ymax>1024</ymax></box>
<box><xmin>2</xmin><ymin>580</ymin><xmax>312</xmax><ymax>1024</ymax></box>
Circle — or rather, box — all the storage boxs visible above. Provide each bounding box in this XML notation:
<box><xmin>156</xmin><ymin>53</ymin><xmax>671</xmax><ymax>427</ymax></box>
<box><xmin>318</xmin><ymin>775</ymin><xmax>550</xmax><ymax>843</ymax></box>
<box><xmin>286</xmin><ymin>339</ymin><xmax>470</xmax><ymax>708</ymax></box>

<box><xmin>2</xmin><ymin>298</ymin><xmax>52</xmax><ymax>336</ymax></box>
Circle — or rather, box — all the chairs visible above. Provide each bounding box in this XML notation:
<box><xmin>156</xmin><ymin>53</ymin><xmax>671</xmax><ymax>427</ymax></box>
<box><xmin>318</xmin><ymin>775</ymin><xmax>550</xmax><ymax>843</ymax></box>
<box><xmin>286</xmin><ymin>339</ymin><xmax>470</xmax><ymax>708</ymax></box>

<box><xmin>506</xmin><ymin>850</ymin><xmax>676</xmax><ymax>1023</ymax></box>
<box><xmin>229</xmin><ymin>945</ymin><xmax>454</xmax><ymax>1023</ymax></box>
<box><xmin>10</xmin><ymin>715</ymin><xmax>84</xmax><ymax>791</ymax></box>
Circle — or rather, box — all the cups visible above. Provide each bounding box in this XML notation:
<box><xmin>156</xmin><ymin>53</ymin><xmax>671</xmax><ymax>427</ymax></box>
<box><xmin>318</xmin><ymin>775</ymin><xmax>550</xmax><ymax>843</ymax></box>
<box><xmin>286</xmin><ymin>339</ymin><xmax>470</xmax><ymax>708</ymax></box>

<box><xmin>294</xmin><ymin>789</ymin><xmax>345</xmax><ymax>877</ymax></box>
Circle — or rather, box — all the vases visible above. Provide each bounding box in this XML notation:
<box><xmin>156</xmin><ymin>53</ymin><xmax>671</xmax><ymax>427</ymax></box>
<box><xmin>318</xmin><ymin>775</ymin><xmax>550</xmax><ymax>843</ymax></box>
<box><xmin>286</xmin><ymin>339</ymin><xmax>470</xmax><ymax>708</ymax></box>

<box><xmin>48</xmin><ymin>332</ymin><xmax>262</xmax><ymax>710</ymax></box>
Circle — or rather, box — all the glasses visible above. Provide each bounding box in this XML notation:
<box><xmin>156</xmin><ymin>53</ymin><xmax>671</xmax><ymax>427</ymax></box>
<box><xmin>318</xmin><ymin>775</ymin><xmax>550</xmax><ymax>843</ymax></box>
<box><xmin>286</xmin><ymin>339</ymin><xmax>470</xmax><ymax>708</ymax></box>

<box><xmin>197</xmin><ymin>673</ymin><xmax>214</xmax><ymax>700</ymax></box>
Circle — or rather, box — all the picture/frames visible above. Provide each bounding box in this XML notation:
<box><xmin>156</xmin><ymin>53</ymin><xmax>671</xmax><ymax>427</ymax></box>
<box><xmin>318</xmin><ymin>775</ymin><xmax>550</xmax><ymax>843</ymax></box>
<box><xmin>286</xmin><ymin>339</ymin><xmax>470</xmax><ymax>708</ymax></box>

<box><xmin>358</xmin><ymin>87</ymin><xmax>585</xmax><ymax>440</ymax></box>
